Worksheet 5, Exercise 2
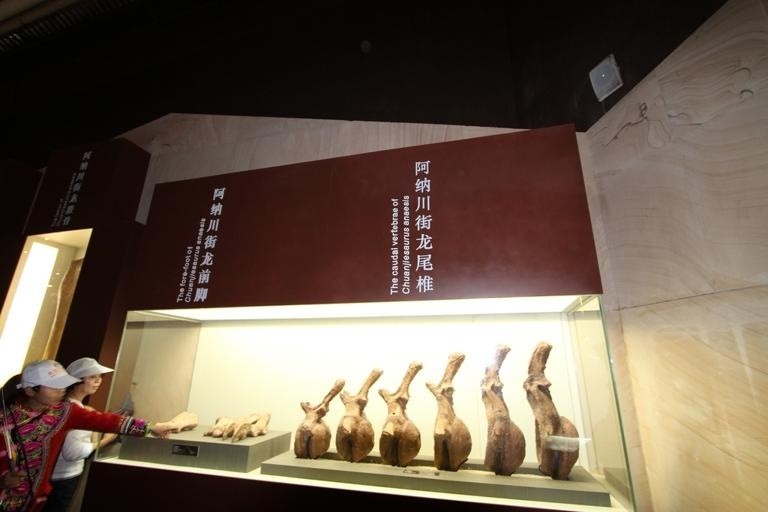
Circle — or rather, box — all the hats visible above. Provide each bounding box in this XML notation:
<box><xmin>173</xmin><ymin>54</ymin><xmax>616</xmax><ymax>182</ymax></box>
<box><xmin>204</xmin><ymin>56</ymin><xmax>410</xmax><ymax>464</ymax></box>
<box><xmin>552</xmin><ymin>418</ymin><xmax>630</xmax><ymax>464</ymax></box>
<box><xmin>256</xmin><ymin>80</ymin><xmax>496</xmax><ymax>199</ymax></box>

<box><xmin>16</xmin><ymin>360</ymin><xmax>83</xmax><ymax>390</ymax></box>
<box><xmin>67</xmin><ymin>357</ymin><xmax>116</xmax><ymax>378</ymax></box>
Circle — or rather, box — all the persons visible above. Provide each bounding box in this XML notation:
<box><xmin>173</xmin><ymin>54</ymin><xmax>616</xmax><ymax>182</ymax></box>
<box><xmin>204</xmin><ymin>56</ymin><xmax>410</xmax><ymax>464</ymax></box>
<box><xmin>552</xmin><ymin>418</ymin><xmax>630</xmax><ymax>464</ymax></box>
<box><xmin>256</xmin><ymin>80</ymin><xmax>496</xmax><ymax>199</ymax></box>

<box><xmin>42</xmin><ymin>356</ymin><xmax>122</xmax><ymax>511</ymax></box>
<box><xmin>0</xmin><ymin>359</ymin><xmax>179</xmax><ymax>510</ymax></box>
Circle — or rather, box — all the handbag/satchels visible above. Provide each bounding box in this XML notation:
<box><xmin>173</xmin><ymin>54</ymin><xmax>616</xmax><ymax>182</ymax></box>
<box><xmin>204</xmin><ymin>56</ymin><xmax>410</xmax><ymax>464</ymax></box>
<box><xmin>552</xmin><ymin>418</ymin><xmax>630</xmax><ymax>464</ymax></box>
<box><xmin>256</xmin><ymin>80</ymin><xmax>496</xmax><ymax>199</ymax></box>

<box><xmin>26</xmin><ymin>497</ymin><xmax>48</xmax><ymax>512</ymax></box>
